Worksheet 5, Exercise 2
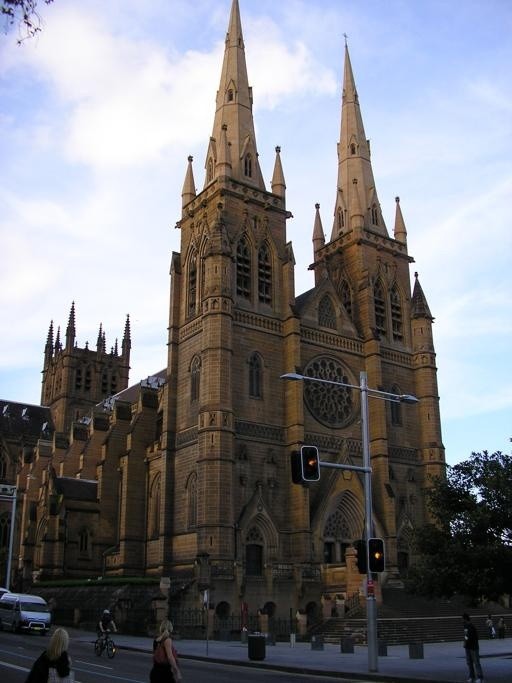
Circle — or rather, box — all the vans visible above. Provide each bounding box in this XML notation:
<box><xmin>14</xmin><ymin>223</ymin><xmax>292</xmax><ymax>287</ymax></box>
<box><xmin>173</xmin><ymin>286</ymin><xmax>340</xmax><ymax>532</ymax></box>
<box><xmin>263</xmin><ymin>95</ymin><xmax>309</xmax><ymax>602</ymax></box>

<box><xmin>1</xmin><ymin>593</ymin><xmax>52</xmax><ymax>636</ymax></box>
<box><xmin>1</xmin><ymin>587</ymin><xmax>12</xmax><ymax>600</ymax></box>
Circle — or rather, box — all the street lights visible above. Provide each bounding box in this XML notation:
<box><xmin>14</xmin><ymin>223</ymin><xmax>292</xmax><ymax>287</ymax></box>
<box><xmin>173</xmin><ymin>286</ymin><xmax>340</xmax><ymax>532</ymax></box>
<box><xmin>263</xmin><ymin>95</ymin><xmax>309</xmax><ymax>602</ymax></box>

<box><xmin>278</xmin><ymin>367</ymin><xmax>426</xmax><ymax>672</ymax></box>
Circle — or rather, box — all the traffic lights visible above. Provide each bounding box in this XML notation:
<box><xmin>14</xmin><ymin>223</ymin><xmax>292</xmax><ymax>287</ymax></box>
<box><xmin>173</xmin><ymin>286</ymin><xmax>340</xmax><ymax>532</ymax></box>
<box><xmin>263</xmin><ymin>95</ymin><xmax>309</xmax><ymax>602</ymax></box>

<box><xmin>300</xmin><ymin>445</ymin><xmax>320</xmax><ymax>483</ymax></box>
<box><xmin>368</xmin><ymin>537</ymin><xmax>386</xmax><ymax>573</ymax></box>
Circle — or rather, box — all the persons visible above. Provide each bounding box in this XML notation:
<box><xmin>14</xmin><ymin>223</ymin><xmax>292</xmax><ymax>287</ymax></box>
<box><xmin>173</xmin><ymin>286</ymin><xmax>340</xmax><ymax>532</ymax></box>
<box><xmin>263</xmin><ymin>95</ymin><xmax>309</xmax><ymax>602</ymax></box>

<box><xmin>486</xmin><ymin>616</ymin><xmax>496</xmax><ymax>639</ymax></box>
<box><xmin>497</xmin><ymin>615</ymin><xmax>505</xmax><ymax>638</ymax></box>
<box><xmin>23</xmin><ymin>626</ymin><xmax>71</xmax><ymax>682</ymax></box>
<box><xmin>95</xmin><ymin>608</ymin><xmax>117</xmax><ymax>648</ymax></box>
<box><xmin>462</xmin><ymin>611</ymin><xmax>485</xmax><ymax>682</ymax></box>
<box><xmin>148</xmin><ymin>618</ymin><xmax>182</xmax><ymax>682</ymax></box>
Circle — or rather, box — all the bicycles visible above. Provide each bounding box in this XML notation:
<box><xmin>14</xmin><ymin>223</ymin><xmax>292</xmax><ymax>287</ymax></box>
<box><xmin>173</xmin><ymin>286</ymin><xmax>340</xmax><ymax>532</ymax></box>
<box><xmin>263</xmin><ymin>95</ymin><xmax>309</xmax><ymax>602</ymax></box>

<box><xmin>94</xmin><ymin>630</ymin><xmax>119</xmax><ymax>659</ymax></box>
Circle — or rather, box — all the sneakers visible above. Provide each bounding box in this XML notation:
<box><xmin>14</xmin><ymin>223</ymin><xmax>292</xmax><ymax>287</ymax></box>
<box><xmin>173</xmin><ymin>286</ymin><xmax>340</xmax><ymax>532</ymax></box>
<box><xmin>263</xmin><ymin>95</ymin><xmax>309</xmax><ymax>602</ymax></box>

<box><xmin>466</xmin><ymin>677</ymin><xmax>485</xmax><ymax>683</ymax></box>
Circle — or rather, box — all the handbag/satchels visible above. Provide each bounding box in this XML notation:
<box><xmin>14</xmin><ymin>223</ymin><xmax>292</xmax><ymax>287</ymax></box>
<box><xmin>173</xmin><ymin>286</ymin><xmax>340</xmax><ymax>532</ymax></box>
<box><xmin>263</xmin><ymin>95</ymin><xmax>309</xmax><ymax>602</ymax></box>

<box><xmin>47</xmin><ymin>667</ymin><xmax>75</xmax><ymax>683</ymax></box>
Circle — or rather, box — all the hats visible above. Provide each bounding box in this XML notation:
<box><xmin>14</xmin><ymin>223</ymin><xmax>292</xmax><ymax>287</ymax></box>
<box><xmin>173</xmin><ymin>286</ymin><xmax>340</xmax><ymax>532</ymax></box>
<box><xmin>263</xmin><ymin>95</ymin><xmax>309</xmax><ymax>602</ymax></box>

<box><xmin>103</xmin><ymin>609</ymin><xmax>110</xmax><ymax>614</ymax></box>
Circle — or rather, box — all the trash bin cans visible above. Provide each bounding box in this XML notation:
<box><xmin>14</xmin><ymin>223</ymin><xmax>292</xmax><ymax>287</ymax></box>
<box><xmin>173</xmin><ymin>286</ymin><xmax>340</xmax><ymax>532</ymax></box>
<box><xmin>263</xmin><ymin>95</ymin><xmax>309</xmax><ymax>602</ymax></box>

<box><xmin>248</xmin><ymin>634</ymin><xmax>265</xmax><ymax>661</ymax></box>
